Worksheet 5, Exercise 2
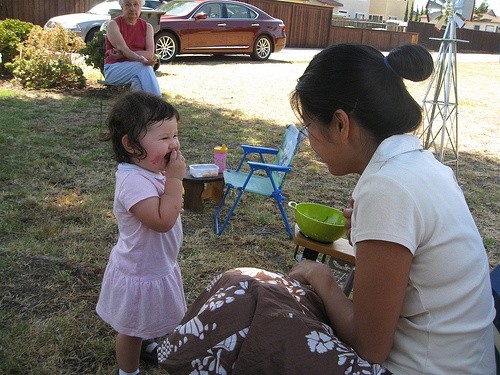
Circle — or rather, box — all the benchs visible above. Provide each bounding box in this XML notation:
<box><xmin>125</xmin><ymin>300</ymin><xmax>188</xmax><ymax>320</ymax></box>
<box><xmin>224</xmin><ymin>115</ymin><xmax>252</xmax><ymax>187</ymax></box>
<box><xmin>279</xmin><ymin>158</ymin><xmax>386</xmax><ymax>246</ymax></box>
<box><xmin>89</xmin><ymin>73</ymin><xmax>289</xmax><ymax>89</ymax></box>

<box><xmin>227</xmin><ymin>12</ymin><xmax>251</xmax><ymax>18</ymax></box>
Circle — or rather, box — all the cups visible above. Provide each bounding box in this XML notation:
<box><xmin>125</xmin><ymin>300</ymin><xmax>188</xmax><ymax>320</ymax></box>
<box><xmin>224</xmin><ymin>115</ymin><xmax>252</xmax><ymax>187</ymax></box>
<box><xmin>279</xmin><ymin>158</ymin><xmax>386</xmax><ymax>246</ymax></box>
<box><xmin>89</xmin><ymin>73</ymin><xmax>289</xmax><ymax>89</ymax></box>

<box><xmin>214</xmin><ymin>144</ymin><xmax>228</xmax><ymax>174</ymax></box>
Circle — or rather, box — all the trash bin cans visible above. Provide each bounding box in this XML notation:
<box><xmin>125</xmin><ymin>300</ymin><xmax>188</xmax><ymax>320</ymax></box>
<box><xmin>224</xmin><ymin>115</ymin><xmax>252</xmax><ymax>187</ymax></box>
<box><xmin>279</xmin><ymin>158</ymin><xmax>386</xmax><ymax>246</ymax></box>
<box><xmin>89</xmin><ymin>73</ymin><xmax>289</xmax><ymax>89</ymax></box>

<box><xmin>386</xmin><ymin>20</ymin><xmax>407</xmax><ymax>33</ymax></box>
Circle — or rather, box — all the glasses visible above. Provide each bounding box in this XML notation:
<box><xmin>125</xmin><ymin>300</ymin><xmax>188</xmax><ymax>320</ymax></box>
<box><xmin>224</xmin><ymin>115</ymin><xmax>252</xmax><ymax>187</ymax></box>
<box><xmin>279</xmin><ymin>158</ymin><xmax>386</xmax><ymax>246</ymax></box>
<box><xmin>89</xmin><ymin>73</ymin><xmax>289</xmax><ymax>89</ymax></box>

<box><xmin>295</xmin><ymin>108</ymin><xmax>351</xmax><ymax>138</ymax></box>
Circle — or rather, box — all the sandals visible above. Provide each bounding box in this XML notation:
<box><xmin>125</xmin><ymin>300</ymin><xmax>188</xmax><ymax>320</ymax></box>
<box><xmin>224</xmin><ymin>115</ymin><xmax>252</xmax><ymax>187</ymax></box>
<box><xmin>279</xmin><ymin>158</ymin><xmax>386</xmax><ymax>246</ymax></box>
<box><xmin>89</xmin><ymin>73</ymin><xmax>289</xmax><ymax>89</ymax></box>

<box><xmin>140</xmin><ymin>338</ymin><xmax>165</xmax><ymax>364</ymax></box>
<box><xmin>111</xmin><ymin>368</ymin><xmax>142</xmax><ymax>375</ymax></box>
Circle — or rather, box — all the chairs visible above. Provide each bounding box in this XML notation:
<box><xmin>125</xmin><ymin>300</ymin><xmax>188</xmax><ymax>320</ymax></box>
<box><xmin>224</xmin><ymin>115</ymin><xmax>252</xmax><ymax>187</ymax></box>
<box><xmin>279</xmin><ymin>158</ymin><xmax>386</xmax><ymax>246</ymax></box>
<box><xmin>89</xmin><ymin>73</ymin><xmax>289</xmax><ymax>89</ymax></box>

<box><xmin>292</xmin><ymin>222</ymin><xmax>500</xmax><ymax>375</ymax></box>
<box><xmin>204</xmin><ymin>6</ymin><xmax>212</xmax><ymax>16</ymax></box>
<box><xmin>215</xmin><ymin>123</ymin><xmax>302</xmax><ymax>238</ymax></box>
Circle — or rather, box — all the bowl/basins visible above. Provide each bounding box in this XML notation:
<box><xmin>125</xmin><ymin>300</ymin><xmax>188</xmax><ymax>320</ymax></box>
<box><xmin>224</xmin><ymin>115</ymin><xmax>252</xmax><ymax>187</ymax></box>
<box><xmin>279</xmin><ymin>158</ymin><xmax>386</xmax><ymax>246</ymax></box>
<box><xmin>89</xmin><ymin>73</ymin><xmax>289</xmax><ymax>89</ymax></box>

<box><xmin>288</xmin><ymin>200</ymin><xmax>349</xmax><ymax>243</ymax></box>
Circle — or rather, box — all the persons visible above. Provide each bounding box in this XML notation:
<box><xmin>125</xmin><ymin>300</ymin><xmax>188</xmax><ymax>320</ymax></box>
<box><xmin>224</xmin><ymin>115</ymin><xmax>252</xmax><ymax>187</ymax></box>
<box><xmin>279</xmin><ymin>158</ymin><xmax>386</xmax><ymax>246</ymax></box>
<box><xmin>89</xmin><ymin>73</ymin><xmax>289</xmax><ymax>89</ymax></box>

<box><xmin>104</xmin><ymin>0</ymin><xmax>161</xmax><ymax>97</ymax></box>
<box><xmin>157</xmin><ymin>42</ymin><xmax>498</xmax><ymax>375</ymax></box>
<box><xmin>96</xmin><ymin>92</ymin><xmax>187</xmax><ymax>375</ymax></box>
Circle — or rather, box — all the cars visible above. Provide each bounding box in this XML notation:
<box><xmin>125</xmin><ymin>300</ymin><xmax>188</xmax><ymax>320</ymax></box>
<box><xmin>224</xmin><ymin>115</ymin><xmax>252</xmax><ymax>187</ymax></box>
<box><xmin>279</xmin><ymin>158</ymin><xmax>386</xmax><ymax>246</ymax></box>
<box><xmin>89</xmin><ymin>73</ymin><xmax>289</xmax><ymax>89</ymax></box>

<box><xmin>43</xmin><ymin>0</ymin><xmax>168</xmax><ymax>55</ymax></box>
<box><xmin>154</xmin><ymin>0</ymin><xmax>287</xmax><ymax>63</ymax></box>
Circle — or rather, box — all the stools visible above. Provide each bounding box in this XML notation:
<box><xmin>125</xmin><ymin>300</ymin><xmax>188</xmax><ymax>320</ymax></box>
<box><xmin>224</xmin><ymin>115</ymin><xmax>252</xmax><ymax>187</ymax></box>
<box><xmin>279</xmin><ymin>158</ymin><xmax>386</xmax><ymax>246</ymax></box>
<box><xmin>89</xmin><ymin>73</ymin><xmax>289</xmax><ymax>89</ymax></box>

<box><xmin>98</xmin><ymin>80</ymin><xmax>132</xmax><ymax>126</ymax></box>
<box><xmin>182</xmin><ymin>171</ymin><xmax>225</xmax><ymax>212</ymax></box>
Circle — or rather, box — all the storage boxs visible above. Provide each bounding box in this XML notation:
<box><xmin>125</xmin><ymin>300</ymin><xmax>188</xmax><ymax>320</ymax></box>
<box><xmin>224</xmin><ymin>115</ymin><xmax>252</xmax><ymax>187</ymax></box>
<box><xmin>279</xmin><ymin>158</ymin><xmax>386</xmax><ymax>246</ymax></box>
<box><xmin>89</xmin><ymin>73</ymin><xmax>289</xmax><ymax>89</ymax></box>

<box><xmin>188</xmin><ymin>164</ymin><xmax>220</xmax><ymax>178</ymax></box>
